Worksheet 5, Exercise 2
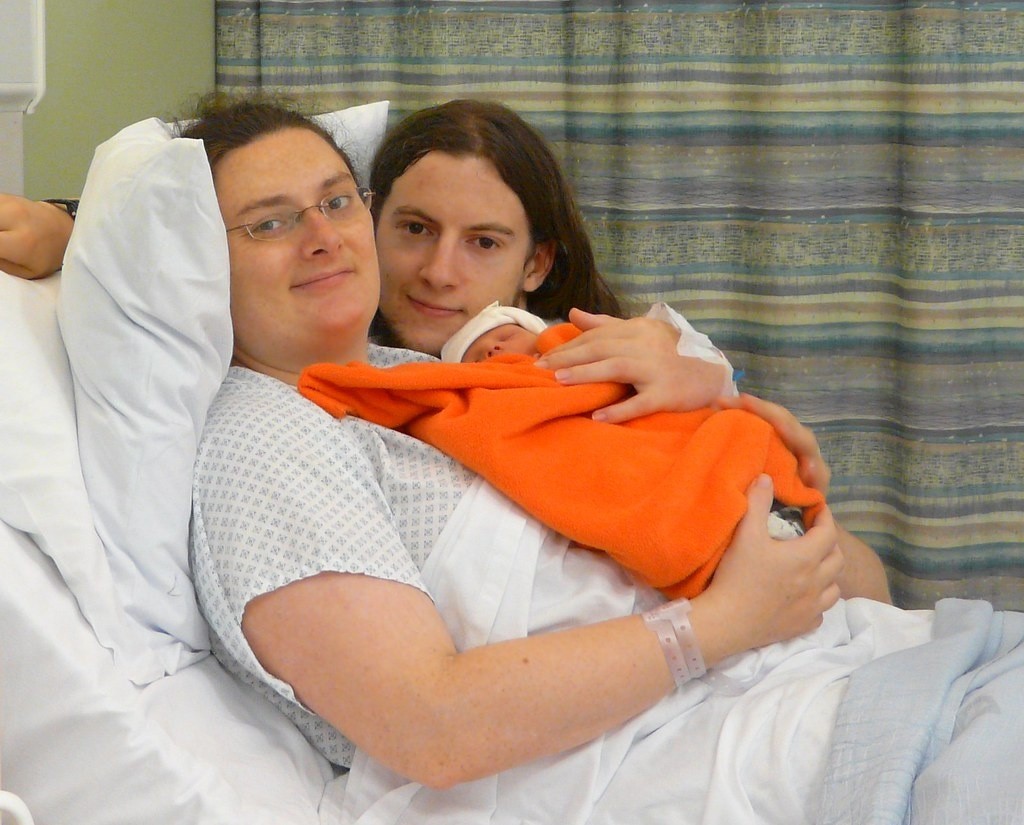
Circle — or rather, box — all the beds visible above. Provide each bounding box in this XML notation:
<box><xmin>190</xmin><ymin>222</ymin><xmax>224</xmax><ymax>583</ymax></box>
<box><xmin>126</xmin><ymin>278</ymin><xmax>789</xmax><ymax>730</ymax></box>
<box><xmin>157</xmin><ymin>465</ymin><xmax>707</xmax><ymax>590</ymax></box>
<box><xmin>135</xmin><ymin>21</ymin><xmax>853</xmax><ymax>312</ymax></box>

<box><xmin>0</xmin><ymin>263</ymin><xmax>1024</xmax><ymax>825</ymax></box>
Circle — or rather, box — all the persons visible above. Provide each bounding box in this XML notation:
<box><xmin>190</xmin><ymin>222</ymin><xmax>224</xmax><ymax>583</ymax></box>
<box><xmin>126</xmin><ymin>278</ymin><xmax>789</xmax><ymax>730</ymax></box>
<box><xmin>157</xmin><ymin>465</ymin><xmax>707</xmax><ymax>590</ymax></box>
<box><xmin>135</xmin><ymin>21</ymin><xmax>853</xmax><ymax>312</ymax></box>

<box><xmin>162</xmin><ymin>93</ymin><xmax>1023</xmax><ymax>825</ymax></box>
<box><xmin>435</xmin><ymin>299</ymin><xmax>826</xmax><ymax>607</ymax></box>
<box><xmin>0</xmin><ymin>95</ymin><xmax>737</xmax><ymax>422</ymax></box>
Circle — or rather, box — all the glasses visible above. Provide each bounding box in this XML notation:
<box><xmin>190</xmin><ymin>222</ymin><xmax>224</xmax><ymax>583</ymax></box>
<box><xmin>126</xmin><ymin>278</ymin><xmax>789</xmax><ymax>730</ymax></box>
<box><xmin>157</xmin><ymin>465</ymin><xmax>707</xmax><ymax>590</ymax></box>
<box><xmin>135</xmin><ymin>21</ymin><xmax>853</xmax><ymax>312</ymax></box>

<box><xmin>223</xmin><ymin>188</ymin><xmax>373</xmax><ymax>243</ymax></box>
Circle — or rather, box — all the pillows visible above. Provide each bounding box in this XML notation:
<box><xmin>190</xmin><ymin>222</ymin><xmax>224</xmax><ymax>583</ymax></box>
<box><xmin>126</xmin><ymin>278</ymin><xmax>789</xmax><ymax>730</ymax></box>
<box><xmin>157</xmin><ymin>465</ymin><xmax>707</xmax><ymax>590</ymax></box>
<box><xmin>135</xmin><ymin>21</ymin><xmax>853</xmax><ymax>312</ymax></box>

<box><xmin>0</xmin><ymin>272</ymin><xmax>213</xmax><ymax>689</ymax></box>
<box><xmin>59</xmin><ymin>98</ymin><xmax>391</xmax><ymax>650</ymax></box>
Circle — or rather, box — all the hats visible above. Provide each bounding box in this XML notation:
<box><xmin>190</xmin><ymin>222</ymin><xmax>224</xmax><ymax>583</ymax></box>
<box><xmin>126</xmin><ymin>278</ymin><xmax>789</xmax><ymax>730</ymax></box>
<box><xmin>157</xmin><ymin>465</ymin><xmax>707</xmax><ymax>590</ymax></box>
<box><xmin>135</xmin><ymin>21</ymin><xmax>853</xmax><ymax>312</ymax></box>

<box><xmin>440</xmin><ymin>301</ymin><xmax>547</xmax><ymax>363</ymax></box>
